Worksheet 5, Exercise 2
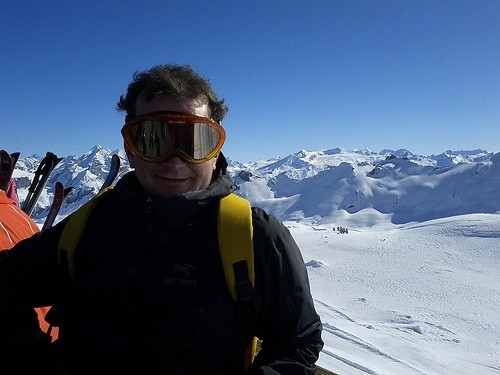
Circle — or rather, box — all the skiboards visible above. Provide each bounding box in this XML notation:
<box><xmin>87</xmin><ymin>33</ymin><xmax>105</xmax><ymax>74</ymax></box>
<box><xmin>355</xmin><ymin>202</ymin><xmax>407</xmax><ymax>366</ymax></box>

<box><xmin>0</xmin><ymin>150</ymin><xmax>121</xmax><ymax>233</ymax></box>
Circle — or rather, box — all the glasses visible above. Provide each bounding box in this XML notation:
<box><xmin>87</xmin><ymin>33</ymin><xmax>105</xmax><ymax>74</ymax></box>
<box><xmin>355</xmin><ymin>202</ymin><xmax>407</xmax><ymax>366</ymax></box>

<box><xmin>120</xmin><ymin>112</ymin><xmax>226</xmax><ymax>164</ymax></box>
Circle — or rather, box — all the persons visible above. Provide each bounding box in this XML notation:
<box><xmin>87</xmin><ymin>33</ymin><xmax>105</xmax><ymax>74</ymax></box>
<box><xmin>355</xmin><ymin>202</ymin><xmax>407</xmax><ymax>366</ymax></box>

<box><xmin>0</xmin><ymin>64</ymin><xmax>326</xmax><ymax>375</ymax></box>
<box><xmin>0</xmin><ymin>149</ymin><xmax>60</xmax><ymax>345</ymax></box>
